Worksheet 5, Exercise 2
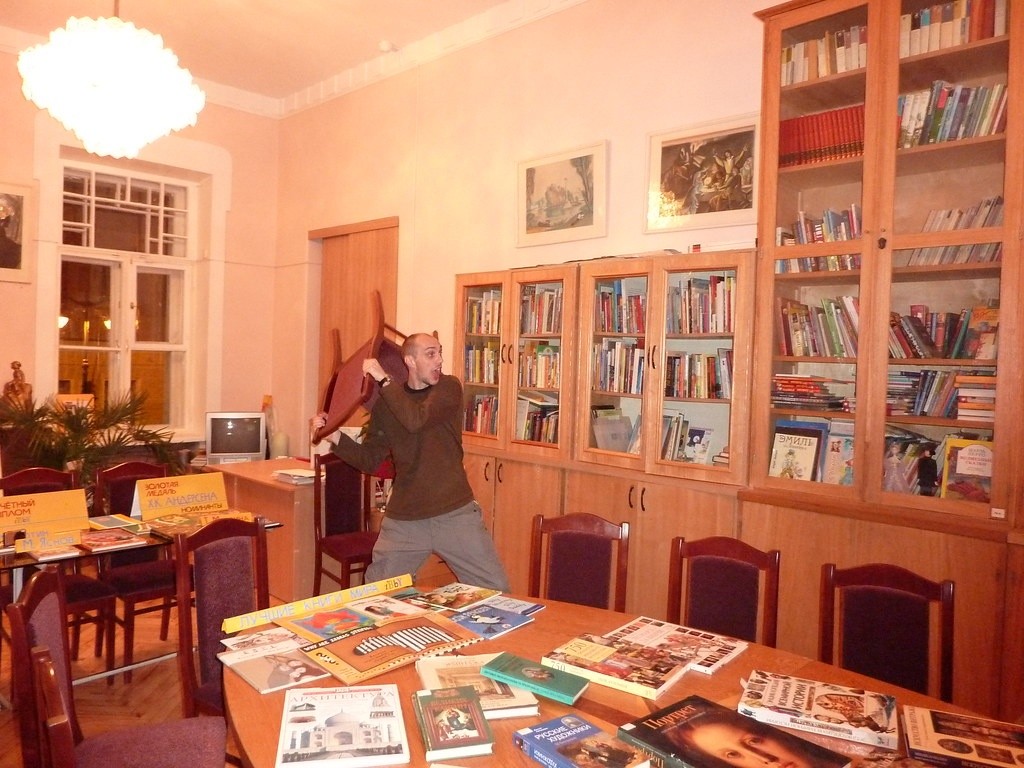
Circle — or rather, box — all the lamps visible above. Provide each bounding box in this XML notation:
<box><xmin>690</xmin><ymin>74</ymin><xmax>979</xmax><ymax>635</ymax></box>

<box><xmin>17</xmin><ymin>0</ymin><xmax>206</xmax><ymax>159</ymax></box>
<box><xmin>59</xmin><ymin>279</ymin><xmax>111</xmax><ymax>335</ymax></box>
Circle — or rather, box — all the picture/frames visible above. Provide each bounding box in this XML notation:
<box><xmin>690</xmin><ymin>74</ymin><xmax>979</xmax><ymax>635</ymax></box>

<box><xmin>645</xmin><ymin>110</ymin><xmax>761</xmax><ymax>233</ymax></box>
<box><xmin>0</xmin><ymin>182</ymin><xmax>33</xmax><ymax>285</ymax></box>
<box><xmin>515</xmin><ymin>139</ymin><xmax>609</xmax><ymax>248</ymax></box>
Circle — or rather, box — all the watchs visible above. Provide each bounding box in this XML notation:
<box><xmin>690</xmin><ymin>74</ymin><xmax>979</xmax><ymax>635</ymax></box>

<box><xmin>378</xmin><ymin>374</ymin><xmax>395</xmax><ymax>389</ymax></box>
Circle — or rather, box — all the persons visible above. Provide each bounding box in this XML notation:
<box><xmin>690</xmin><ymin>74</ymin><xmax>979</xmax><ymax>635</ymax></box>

<box><xmin>914</xmin><ymin>447</ymin><xmax>938</xmax><ymax>496</ymax></box>
<box><xmin>308</xmin><ymin>333</ymin><xmax>510</xmax><ymax>594</ymax></box>
<box><xmin>883</xmin><ymin>441</ymin><xmax>913</xmax><ymax>493</ymax></box>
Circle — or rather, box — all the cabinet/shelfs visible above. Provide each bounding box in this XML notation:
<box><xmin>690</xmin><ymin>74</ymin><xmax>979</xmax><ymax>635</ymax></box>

<box><xmin>205</xmin><ymin>458</ymin><xmax>360</xmax><ymax>603</ymax></box>
<box><xmin>415</xmin><ymin>1</ymin><xmax>1024</xmax><ymax>728</ymax></box>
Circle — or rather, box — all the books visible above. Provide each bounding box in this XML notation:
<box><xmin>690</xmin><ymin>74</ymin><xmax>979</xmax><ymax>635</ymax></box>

<box><xmin>882</xmin><ymin>364</ymin><xmax>997</xmax><ymax>502</ymax></box>
<box><xmin>591</xmin><ymin>276</ymin><xmax>648</xmax><ymax>456</ymax></box>
<box><xmin>25</xmin><ymin>513</ymin><xmax>200</xmax><ymax>563</ymax></box>
<box><xmin>215</xmin><ymin>579</ymin><xmax>1024</xmax><ymax>768</ymax></box>
<box><xmin>778</xmin><ymin>80</ymin><xmax>866</xmax><ymax>167</ymax></box>
<box><xmin>906</xmin><ymin>193</ymin><xmax>1003</xmax><ymax>265</ymax></box>
<box><xmin>661</xmin><ymin>271</ymin><xmax>737</xmax><ymax>471</ymax></box>
<box><xmin>897</xmin><ymin>80</ymin><xmax>1007</xmax><ymax>147</ymax></box>
<box><xmin>462</xmin><ymin>286</ymin><xmax>503</xmax><ymax>436</ymax></box>
<box><xmin>780</xmin><ymin>0</ymin><xmax>867</xmax><ymax>87</ymax></box>
<box><xmin>768</xmin><ymin>363</ymin><xmax>856</xmax><ymax>483</ymax></box>
<box><xmin>516</xmin><ymin>283</ymin><xmax>561</xmax><ymax>446</ymax></box>
<box><xmin>888</xmin><ymin>305</ymin><xmax>999</xmax><ymax>360</ymax></box>
<box><xmin>272</xmin><ymin>468</ymin><xmax>327</xmax><ymax>485</ymax></box>
<box><xmin>898</xmin><ymin>0</ymin><xmax>1009</xmax><ymax>59</ymax></box>
<box><xmin>775</xmin><ymin>195</ymin><xmax>864</xmax><ymax>274</ymax></box>
<box><xmin>773</xmin><ymin>295</ymin><xmax>859</xmax><ymax>358</ymax></box>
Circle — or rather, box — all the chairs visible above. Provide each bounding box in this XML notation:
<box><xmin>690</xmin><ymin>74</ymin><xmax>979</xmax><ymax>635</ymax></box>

<box><xmin>6</xmin><ymin>564</ymin><xmax>228</xmax><ymax>768</ymax></box>
<box><xmin>666</xmin><ymin>536</ymin><xmax>781</xmax><ymax>649</ymax></box>
<box><xmin>527</xmin><ymin>511</ymin><xmax>630</xmax><ymax>614</ymax></box>
<box><xmin>313</xmin><ymin>452</ymin><xmax>379</xmax><ymax>597</ymax></box>
<box><xmin>818</xmin><ymin>562</ymin><xmax>955</xmax><ymax>706</ymax></box>
<box><xmin>95</xmin><ymin>461</ymin><xmax>196</xmax><ymax>683</ymax></box>
<box><xmin>0</xmin><ymin>467</ymin><xmax>118</xmax><ymax>686</ymax></box>
<box><xmin>30</xmin><ymin>645</ymin><xmax>79</xmax><ymax>768</ymax></box>
<box><xmin>174</xmin><ymin>515</ymin><xmax>270</xmax><ymax>768</ymax></box>
<box><xmin>308</xmin><ymin>289</ymin><xmax>439</xmax><ymax>448</ymax></box>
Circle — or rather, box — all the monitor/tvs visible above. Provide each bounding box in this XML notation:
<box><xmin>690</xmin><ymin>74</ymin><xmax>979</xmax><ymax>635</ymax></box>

<box><xmin>206</xmin><ymin>411</ymin><xmax>266</xmax><ymax>465</ymax></box>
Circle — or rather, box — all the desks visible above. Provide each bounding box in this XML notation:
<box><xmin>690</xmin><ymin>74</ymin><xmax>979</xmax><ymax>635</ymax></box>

<box><xmin>222</xmin><ymin>586</ymin><xmax>991</xmax><ymax>768</ymax></box>
<box><xmin>0</xmin><ymin>508</ymin><xmax>285</xmax><ymax>713</ymax></box>
